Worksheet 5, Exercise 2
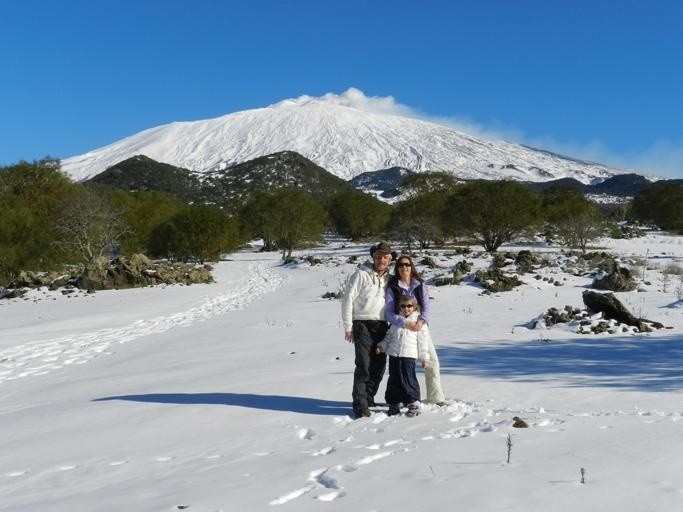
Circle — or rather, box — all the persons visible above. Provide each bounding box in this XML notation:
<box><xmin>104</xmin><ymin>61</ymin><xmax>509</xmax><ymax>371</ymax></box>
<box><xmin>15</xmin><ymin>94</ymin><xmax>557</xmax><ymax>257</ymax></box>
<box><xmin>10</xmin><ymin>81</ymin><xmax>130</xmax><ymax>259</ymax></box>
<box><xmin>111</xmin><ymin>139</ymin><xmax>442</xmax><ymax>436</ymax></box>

<box><xmin>375</xmin><ymin>295</ymin><xmax>430</xmax><ymax>418</ymax></box>
<box><xmin>339</xmin><ymin>242</ymin><xmax>394</xmax><ymax>419</ymax></box>
<box><xmin>383</xmin><ymin>255</ymin><xmax>447</xmax><ymax>408</ymax></box>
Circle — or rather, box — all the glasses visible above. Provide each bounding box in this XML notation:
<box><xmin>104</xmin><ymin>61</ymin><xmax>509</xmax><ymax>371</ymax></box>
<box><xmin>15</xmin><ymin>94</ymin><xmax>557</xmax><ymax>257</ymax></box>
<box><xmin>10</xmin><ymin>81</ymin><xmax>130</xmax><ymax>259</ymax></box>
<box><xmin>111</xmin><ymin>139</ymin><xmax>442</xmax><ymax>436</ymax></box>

<box><xmin>400</xmin><ymin>303</ymin><xmax>413</xmax><ymax>308</ymax></box>
<box><xmin>397</xmin><ymin>262</ymin><xmax>411</xmax><ymax>269</ymax></box>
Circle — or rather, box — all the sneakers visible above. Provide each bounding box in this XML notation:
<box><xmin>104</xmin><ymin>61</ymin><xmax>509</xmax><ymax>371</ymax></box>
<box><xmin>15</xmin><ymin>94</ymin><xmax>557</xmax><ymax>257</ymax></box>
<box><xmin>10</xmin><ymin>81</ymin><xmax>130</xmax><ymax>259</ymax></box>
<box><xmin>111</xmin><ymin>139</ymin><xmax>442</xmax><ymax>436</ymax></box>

<box><xmin>352</xmin><ymin>401</ymin><xmax>383</xmax><ymax>417</ymax></box>
<box><xmin>406</xmin><ymin>404</ymin><xmax>419</xmax><ymax>419</ymax></box>
<box><xmin>385</xmin><ymin>401</ymin><xmax>401</xmax><ymax>416</ymax></box>
<box><xmin>435</xmin><ymin>400</ymin><xmax>447</xmax><ymax>407</ymax></box>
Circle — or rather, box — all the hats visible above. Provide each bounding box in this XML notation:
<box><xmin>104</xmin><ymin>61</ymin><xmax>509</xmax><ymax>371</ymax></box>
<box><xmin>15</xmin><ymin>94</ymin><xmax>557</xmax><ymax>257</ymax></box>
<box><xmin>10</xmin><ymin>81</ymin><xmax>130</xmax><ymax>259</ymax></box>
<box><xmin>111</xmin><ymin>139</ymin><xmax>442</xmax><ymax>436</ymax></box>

<box><xmin>369</xmin><ymin>240</ymin><xmax>396</xmax><ymax>260</ymax></box>
